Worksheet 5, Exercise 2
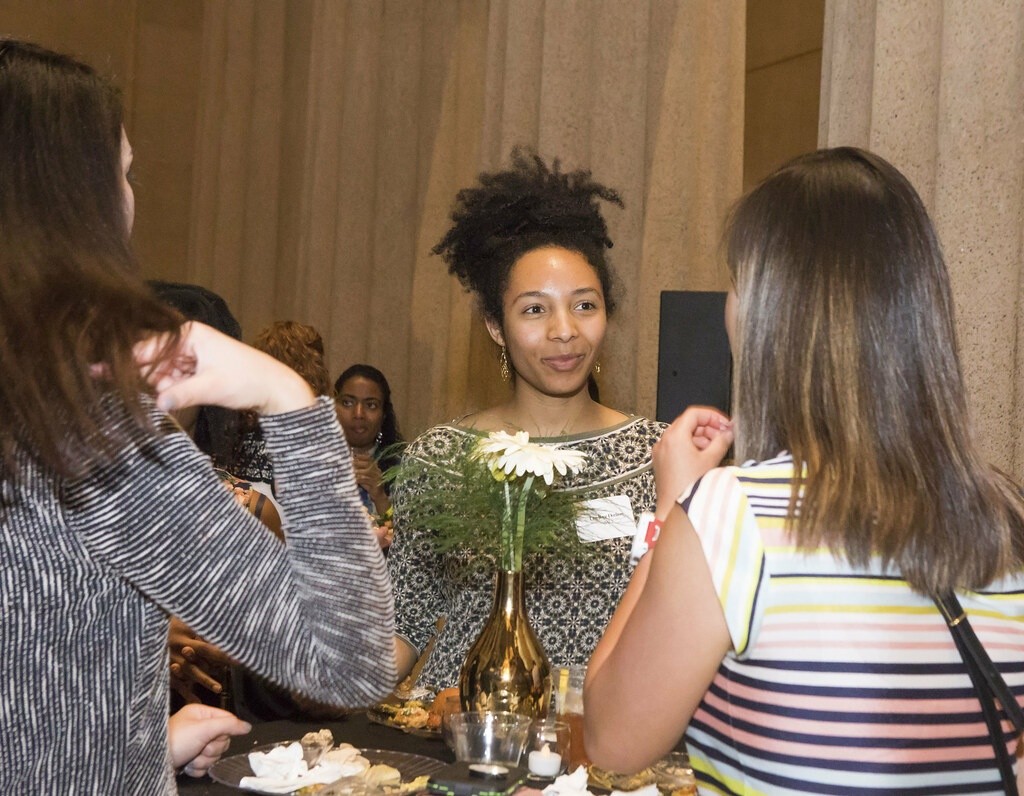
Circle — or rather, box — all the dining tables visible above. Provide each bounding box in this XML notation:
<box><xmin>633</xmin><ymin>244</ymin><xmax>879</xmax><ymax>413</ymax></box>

<box><xmin>174</xmin><ymin>719</ymin><xmax>562</xmax><ymax>796</ymax></box>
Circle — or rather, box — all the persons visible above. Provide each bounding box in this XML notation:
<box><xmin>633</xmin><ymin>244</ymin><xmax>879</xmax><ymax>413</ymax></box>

<box><xmin>584</xmin><ymin>145</ymin><xmax>1024</xmax><ymax>796</ymax></box>
<box><xmin>232</xmin><ymin>321</ymin><xmax>325</xmax><ymax>480</ymax></box>
<box><xmin>0</xmin><ymin>37</ymin><xmax>401</xmax><ymax>796</ymax></box>
<box><xmin>385</xmin><ymin>145</ymin><xmax>671</xmax><ymax>717</ymax></box>
<box><xmin>332</xmin><ymin>364</ymin><xmax>409</xmax><ymax>519</ymax></box>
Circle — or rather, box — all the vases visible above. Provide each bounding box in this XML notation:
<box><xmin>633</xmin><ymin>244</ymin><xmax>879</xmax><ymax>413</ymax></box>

<box><xmin>455</xmin><ymin>572</ymin><xmax>555</xmax><ymax>736</ymax></box>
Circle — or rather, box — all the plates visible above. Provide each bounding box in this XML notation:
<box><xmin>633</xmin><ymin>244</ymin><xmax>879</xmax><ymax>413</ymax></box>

<box><xmin>207</xmin><ymin>747</ymin><xmax>451</xmax><ymax>796</ymax></box>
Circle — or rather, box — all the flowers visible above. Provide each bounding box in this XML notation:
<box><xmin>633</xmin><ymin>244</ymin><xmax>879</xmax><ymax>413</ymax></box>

<box><xmin>369</xmin><ymin>396</ymin><xmax>641</xmax><ymax>576</ymax></box>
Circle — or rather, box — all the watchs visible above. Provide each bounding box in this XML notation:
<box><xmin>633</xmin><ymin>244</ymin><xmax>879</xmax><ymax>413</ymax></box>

<box><xmin>629</xmin><ymin>513</ymin><xmax>663</xmax><ymax>565</ymax></box>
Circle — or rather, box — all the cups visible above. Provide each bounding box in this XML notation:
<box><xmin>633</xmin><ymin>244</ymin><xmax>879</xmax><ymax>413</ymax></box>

<box><xmin>551</xmin><ymin>664</ymin><xmax>591</xmax><ymax>774</ymax></box>
<box><xmin>532</xmin><ymin>719</ymin><xmax>574</xmax><ymax>775</ymax></box>
<box><xmin>449</xmin><ymin>711</ymin><xmax>533</xmax><ymax>769</ymax></box>
